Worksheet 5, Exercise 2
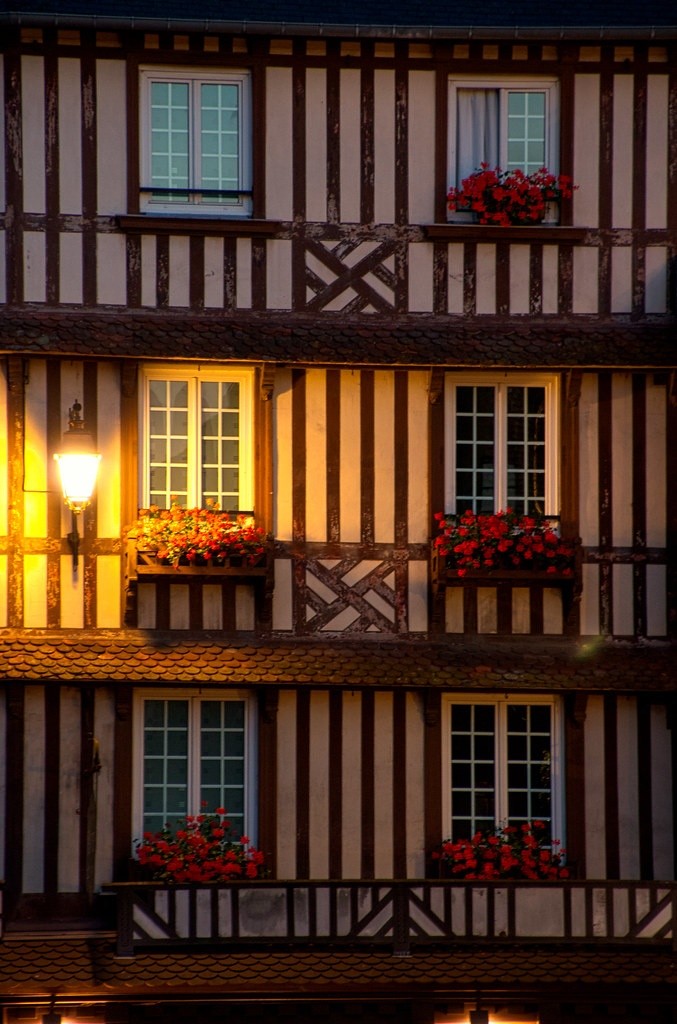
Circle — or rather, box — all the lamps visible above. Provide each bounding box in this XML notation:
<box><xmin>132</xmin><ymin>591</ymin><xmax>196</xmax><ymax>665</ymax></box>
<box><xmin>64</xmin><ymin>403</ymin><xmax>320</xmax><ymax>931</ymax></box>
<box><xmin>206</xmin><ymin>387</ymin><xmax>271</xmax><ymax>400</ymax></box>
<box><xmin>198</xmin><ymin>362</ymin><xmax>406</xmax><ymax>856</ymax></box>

<box><xmin>54</xmin><ymin>399</ymin><xmax>103</xmax><ymax>571</ymax></box>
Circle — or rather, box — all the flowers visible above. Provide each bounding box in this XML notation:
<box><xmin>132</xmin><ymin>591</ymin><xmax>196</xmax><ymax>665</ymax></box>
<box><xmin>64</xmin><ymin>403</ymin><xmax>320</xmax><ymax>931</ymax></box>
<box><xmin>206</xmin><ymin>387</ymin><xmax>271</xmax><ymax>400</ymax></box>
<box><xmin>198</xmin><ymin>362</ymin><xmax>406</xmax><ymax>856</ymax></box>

<box><xmin>130</xmin><ymin>801</ymin><xmax>268</xmax><ymax>884</ymax></box>
<box><xmin>431</xmin><ymin>506</ymin><xmax>577</xmax><ymax>577</ymax></box>
<box><xmin>433</xmin><ymin>818</ymin><xmax>569</xmax><ymax>880</ymax></box>
<box><xmin>129</xmin><ymin>494</ymin><xmax>270</xmax><ymax>571</ymax></box>
<box><xmin>447</xmin><ymin>161</ymin><xmax>581</xmax><ymax>227</ymax></box>
